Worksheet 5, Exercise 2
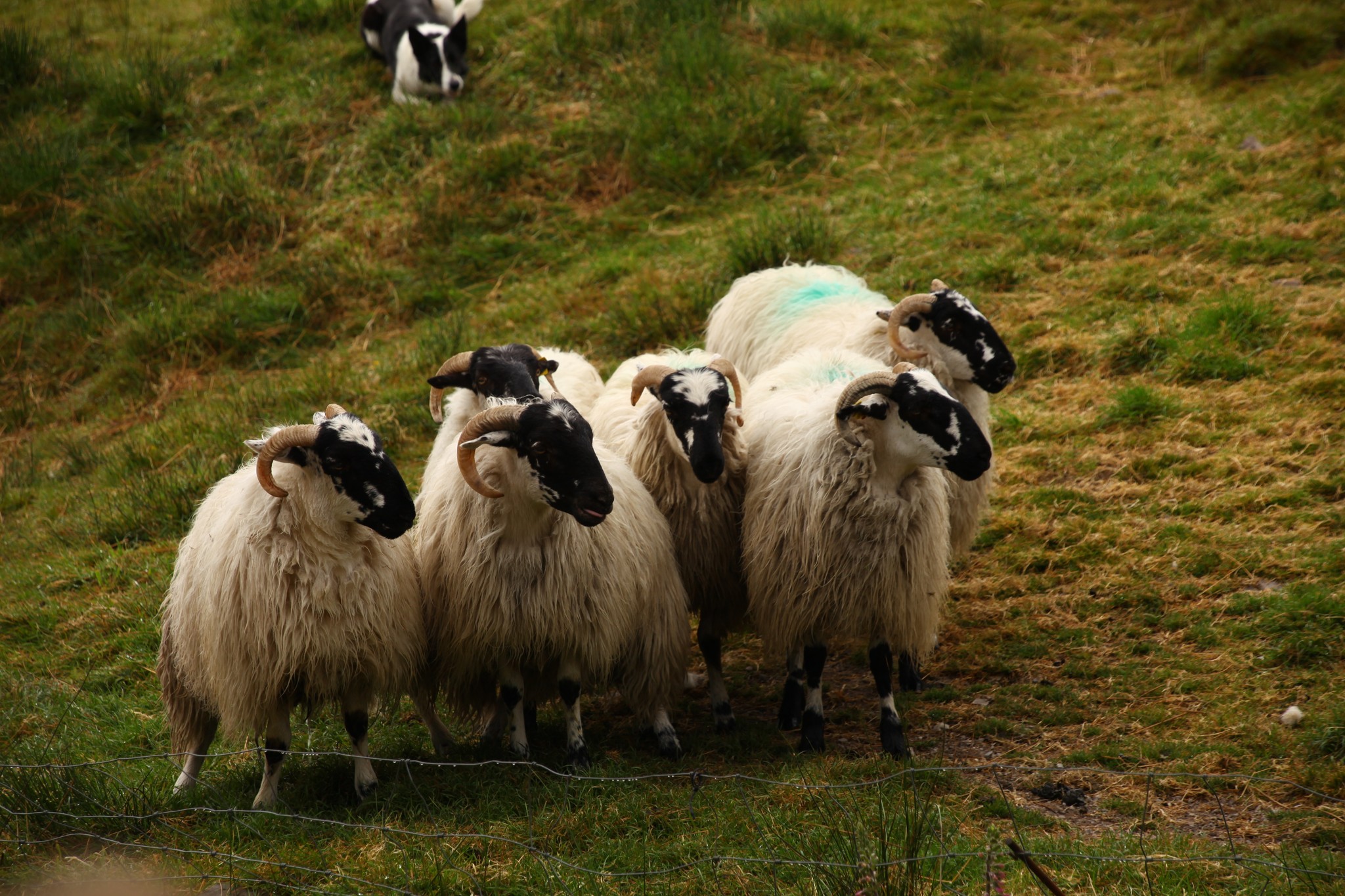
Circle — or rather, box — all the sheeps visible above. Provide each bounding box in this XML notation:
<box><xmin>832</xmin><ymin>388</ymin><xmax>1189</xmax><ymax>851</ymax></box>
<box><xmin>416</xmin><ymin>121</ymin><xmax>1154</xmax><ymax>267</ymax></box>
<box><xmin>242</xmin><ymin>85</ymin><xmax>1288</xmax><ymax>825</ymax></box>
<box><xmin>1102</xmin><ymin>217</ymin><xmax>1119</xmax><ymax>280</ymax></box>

<box><xmin>404</xmin><ymin>388</ymin><xmax>701</xmax><ymax>764</ymax></box>
<box><xmin>585</xmin><ymin>341</ymin><xmax>763</xmax><ymax>707</ymax></box>
<box><xmin>738</xmin><ymin>339</ymin><xmax>996</xmax><ymax>769</ymax></box>
<box><xmin>422</xmin><ymin>334</ymin><xmax>608</xmax><ymax>431</ymax></box>
<box><xmin>691</xmin><ymin>252</ymin><xmax>1018</xmax><ymax>562</ymax></box>
<box><xmin>141</xmin><ymin>396</ymin><xmax>465</xmax><ymax>812</ymax></box>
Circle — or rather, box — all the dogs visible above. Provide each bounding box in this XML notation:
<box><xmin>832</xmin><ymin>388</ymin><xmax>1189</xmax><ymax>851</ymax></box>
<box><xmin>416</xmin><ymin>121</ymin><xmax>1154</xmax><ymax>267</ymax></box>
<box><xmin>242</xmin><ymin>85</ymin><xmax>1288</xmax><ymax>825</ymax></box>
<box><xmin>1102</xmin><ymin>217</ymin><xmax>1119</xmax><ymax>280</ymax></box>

<box><xmin>356</xmin><ymin>0</ymin><xmax>486</xmax><ymax>110</ymax></box>
